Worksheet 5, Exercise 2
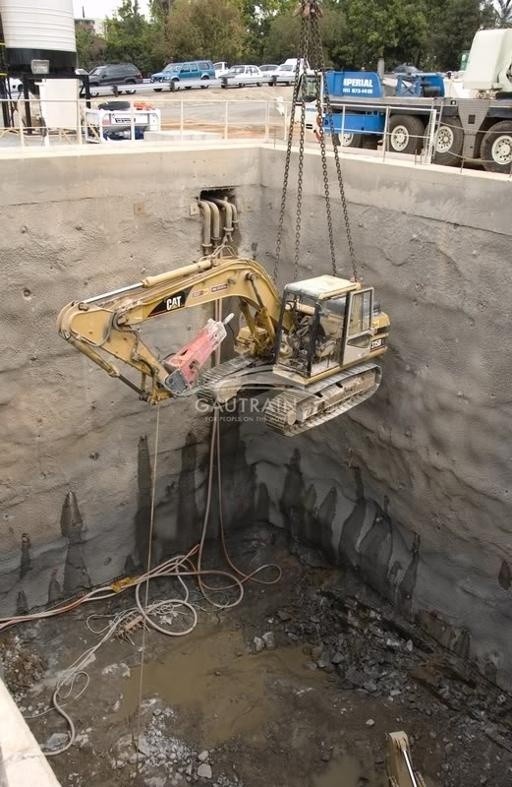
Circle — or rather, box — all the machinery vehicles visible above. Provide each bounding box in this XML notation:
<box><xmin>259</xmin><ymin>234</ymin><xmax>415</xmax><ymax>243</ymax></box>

<box><xmin>385</xmin><ymin>730</ymin><xmax>426</xmax><ymax>787</ymax></box>
<box><xmin>56</xmin><ymin>251</ymin><xmax>392</xmax><ymax>440</ymax></box>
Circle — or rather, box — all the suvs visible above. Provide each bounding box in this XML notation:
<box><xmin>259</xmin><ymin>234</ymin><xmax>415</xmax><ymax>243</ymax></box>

<box><xmin>150</xmin><ymin>59</ymin><xmax>215</xmax><ymax>92</ymax></box>
<box><xmin>80</xmin><ymin>62</ymin><xmax>143</xmax><ymax>97</ymax></box>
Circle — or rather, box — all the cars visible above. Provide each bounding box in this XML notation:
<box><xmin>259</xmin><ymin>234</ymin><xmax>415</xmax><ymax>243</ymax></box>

<box><xmin>391</xmin><ymin>65</ymin><xmax>424</xmax><ymax>73</ymax></box>
<box><xmin>3</xmin><ymin>75</ymin><xmax>24</xmax><ymax>93</ymax></box>
<box><xmin>214</xmin><ymin>62</ymin><xmax>301</xmax><ymax>88</ymax></box>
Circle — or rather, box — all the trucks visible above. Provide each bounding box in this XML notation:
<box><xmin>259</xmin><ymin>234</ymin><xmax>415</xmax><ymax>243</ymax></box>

<box><xmin>293</xmin><ymin>27</ymin><xmax>512</xmax><ymax>174</ymax></box>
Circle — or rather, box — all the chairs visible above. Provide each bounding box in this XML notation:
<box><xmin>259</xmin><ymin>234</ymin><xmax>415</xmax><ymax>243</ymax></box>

<box><xmin>299</xmin><ymin>339</ymin><xmax>337</xmax><ymax>369</ymax></box>
